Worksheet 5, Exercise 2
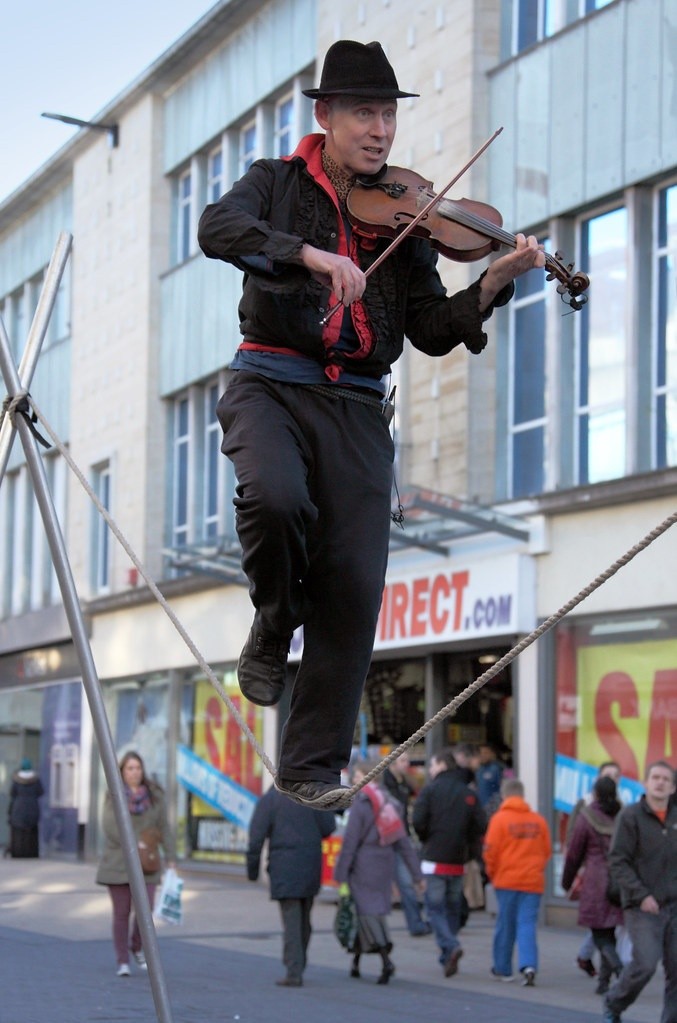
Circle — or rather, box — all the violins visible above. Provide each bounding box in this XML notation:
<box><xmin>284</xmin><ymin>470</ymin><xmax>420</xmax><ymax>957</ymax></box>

<box><xmin>343</xmin><ymin>160</ymin><xmax>590</xmax><ymax>308</ymax></box>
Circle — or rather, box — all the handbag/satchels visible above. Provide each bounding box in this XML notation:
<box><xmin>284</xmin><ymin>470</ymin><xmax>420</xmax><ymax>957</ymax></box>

<box><xmin>334</xmin><ymin>896</ymin><xmax>358</xmax><ymax>952</ymax></box>
<box><xmin>157</xmin><ymin>868</ymin><xmax>184</xmax><ymax>926</ymax></box>
<box><xmin>138</xmin><ymin>832</ymin><xmax>160</xmax><ymax>874</ymax></box>
<box><xmin>605</xmin><ymin>882</ymin><xmax>622</xmax><ymax>907</ymax></box>
<box><xmin>463</xmin><ymin>861</ymin><xmax>484</xmax><ymax>908</ymax></box>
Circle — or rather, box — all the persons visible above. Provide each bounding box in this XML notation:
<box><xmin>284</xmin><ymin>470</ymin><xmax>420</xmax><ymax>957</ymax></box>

<box><xmin>560</xmin><ymin>775</ymin><xmax>625</xmax><ymax>996</ymax></box>
<box><xmin>7</xmin><ymin>758</ymin><xmax>45</xmax><ymax>858</ymax></box>
<box><xmin>482</xmin><ymin>778</ymin><xmax>552</xmax><ymax>987</ymax></box>
<box><xmin>333</xmin><ymin>759</ymin><xmax>428</xmax><ymax>985</ymax></box>
<box><xmin>245</xmin><ymin>781</ymin><xmax>337</xmax><ymax>987</ymax></box>
<box><xmin>245</xmin><ymin>743</ymin><xmax>677</xmax><ymax>1023</ymax></box>
<box><xmin>95</xmin><ymin>750</ymin><xmax>177</xmax><ymax>976</ymax></box>
<box><xmin>197</xmin><ymin>39</ymin><xmax>546</xmax><ymax>811</ymax></box>
<box><xmin>602</xmin><ymin>759</ymin><xmax>677</xmax><ymax>1023</ymax></box>
<box><xmin>412</xmin><ymin>750</ymin><xmax>488</xmax><ymax>978</ymax></box>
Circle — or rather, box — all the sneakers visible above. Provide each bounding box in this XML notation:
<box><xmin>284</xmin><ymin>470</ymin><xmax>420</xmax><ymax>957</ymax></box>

<box><xmin>116</xmin><ymin>963</ymin><xmax>131</xmax><ymax>976</ymax></box>
<box><xmin>128</xmin><ymin>947</ymin><xmax>147</xmax><ymax>970</ymax></box>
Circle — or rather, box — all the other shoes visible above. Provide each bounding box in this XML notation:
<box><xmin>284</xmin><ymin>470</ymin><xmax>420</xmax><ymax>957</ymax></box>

<box><xmin>602</xmin><ymin>1000</ymin><xmax>623</xmax><ymax>1023</ymax></box>
<box><xmin>491</xmin><ymin>967</ymin><xmax>515</xmax><ymax>982</ymax></box>
<box><xmin>576</xmin><ymin>958</ymin><xmax>595</xmax><ymax>976</ymax></box>
<box><xmin>377</xmin><ymin>963</ymin><xmax>395</xmax><ymax>985</ymax></box>
<box><xmin>411</xmin><ymin>922</ymin><xmax>433</xmax><ymax>936</ymax></box>
<box><xmin>350</xmin><ymin>968</ymin><xmax>360</xmax><ymax>978</ymax></box>
<box><xmin>596</xmin><ymin>984</ymin><xmax>608</xmax><ymax>994</ymax></box>
<box><xmin>522</xmin><ymin>967</ymin><xmax>536</xmax><ymax>986</ymax></box>
<box><xmin>444</xmin><ymin>947</ymin><xmax>463</xmax><ymax>976</ymax></box>
<box><xmin>275</xmin><ymin>978</ymin><xmax>304</xmax><ymax>986</ymax></box>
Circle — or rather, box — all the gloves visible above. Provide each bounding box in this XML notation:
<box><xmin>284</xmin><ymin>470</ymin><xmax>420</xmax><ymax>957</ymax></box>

<box><xmin>338</xmin><ymin>882</ymin><xmax>352</xmax><ymax>897</ymax></box>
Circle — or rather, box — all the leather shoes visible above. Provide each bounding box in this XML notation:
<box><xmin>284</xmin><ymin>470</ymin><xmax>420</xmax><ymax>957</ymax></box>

<box><xmin>238</xmin><ymin>610</ymin><xmax>293</xmax><ymax>705</ymax></box>
<box><xmin>275</xmin><ymin>775</ymin><xmax>354</xmax><ymax>810</ymax></box>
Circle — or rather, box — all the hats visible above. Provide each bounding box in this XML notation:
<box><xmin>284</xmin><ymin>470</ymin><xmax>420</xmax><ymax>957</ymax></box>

<box><xmin>21</xmin><ymin>759</ymin><xmax>32</xmax><ymax>768</ymax></box>
<box><xmin>302</xmin><ymin>40</ymin><xmax>421</xmax><ymax>100</ymax></box>
